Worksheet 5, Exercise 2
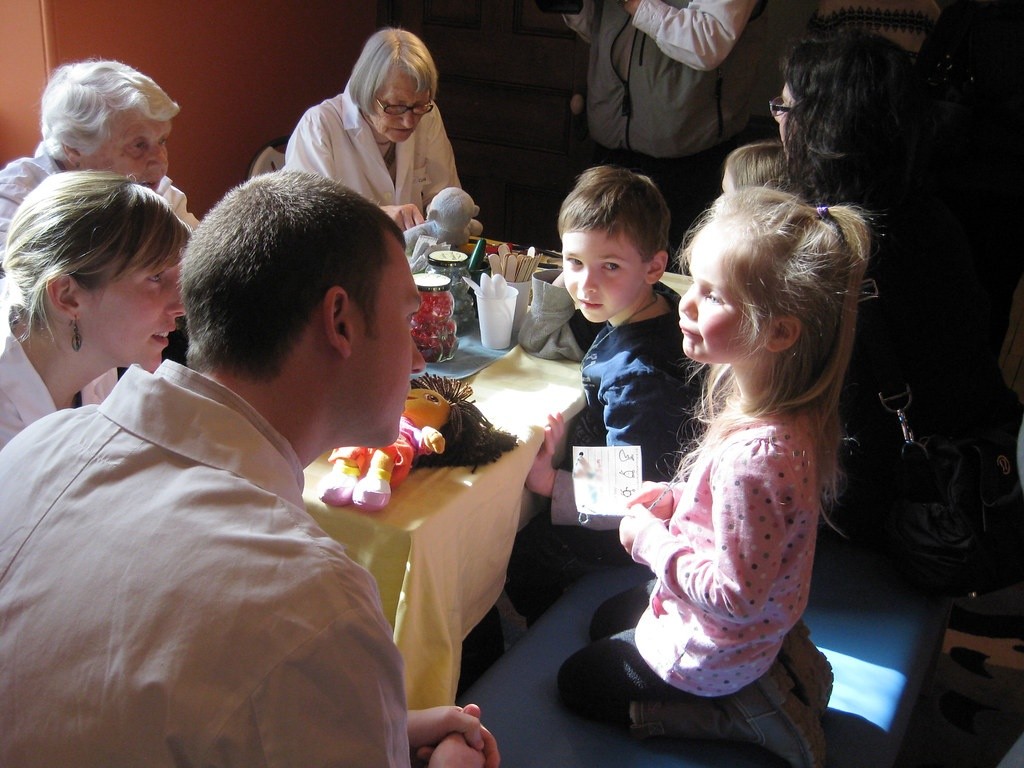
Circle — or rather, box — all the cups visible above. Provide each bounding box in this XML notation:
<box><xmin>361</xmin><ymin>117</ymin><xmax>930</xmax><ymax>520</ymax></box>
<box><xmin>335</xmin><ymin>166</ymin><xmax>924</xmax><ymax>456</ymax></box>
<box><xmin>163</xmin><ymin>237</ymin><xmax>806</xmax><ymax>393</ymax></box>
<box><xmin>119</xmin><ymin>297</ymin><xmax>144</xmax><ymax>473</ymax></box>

<box><xmin>474</xmin><ymin>284</ymin><xmax>519</xmax><ymax>349</ymax></box>
<box><xmin>503</xmin><ymin>281</ymin><xmax>531</xmax><ymax>332</ymax></box>
<box><xmin>466</xmin><ymin>260</ymin><xmax>491</xmax><ymax>319</ymax></box>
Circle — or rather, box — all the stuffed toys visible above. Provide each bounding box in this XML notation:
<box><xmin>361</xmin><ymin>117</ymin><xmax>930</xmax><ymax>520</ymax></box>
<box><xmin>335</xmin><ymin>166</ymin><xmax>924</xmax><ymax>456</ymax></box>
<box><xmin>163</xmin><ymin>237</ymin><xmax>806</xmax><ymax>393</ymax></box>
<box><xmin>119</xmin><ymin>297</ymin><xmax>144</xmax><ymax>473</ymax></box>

<box><xmin>403</xmin><ymin>187</ymin><xmax>483</xmax><ymax>258</ymax></box>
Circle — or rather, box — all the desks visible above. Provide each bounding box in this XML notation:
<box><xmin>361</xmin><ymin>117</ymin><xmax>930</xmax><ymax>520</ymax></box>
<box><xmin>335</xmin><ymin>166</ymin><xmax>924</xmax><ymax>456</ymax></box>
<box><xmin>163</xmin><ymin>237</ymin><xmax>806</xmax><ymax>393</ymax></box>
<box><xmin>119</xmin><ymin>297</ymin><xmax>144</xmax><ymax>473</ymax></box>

<box><xmin>300</xmin><ymin>236</ymin><xmax>694</xmax><ymax>710</ymax></box>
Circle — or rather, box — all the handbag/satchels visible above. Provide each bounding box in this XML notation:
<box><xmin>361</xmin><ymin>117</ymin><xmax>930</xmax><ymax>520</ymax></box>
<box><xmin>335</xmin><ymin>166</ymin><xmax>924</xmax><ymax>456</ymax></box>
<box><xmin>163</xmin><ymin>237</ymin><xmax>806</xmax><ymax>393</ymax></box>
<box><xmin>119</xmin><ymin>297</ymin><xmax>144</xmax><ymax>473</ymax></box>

<box><xmin>883</xmin><ymin>420</ymin><xmax>1024</xmax><ymax>596</ymax></box>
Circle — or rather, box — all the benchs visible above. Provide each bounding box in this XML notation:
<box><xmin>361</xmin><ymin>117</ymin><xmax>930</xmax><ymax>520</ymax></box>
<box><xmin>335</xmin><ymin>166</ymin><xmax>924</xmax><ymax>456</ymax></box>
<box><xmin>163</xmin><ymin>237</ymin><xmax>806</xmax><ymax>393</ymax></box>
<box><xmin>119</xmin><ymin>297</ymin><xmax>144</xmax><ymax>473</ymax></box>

<box><xmin>455</xmin><ymin>562</ymin><xmax>950</xmax><ymax>768</ymax></box>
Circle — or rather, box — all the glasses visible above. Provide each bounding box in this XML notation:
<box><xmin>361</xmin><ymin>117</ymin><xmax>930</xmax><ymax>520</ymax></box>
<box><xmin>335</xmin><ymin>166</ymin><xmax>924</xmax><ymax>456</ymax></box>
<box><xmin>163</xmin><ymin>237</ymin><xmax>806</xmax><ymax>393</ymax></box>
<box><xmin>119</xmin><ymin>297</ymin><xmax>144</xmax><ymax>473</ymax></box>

<box><xmin>376</xmin><ymin>98</ymin><xmax>434</xmax><ymax>115</ymax></box>
<box><xmin>769</xmin><ymin>96</ymin><xmax>794</xmax><ymax>117</ymax></box>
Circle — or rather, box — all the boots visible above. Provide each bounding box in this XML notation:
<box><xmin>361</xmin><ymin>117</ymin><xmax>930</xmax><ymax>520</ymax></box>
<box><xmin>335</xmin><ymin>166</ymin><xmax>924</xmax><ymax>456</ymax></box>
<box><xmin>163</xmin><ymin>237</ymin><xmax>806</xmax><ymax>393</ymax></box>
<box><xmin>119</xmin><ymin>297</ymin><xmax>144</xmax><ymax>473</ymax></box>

<box><xmin>630</xmin><ymin>617</ymin><xmax>834</xmax><ymax>768</ymax></box>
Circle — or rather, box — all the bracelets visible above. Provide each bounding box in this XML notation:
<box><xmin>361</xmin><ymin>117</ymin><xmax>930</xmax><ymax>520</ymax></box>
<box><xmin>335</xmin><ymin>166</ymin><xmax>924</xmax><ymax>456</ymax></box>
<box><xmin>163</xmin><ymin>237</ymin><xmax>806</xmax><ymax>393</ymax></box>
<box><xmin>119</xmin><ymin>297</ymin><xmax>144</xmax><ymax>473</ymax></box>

<box><xmin>616</xmin><ymin>0</ymin><xmax>628</xmax><ymax>8</ymax></box>
<box><xmin>645</xmin><ymin>471</ymin><xmax>685</xmax><ymax>512</ymax></box>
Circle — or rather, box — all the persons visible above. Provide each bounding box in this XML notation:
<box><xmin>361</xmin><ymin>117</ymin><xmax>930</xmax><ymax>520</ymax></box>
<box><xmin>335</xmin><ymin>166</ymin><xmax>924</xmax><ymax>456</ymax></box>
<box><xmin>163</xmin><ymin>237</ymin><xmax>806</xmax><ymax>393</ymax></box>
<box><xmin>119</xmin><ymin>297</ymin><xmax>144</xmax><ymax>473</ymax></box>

<box><xmin>319</xmin><ymin>371</ymin><xmax>518</xmax><ymax>512</ymax></box>
<box><xmin>285</xmin><ymin>27</ymin><xmax>462</xmax><ymax>232</ymax></box>
<box><xmin>525</xmin><ymin>186</ymin><xmax>870</xmax><ymax>768</ymax></box>
<box><xmin>563</xmin><ymin>0</ymin><xmax>767</xmax><ymax>249</ymax></box>
<box><xmin>506</xmin><ymin>167</ymin><xmax>712</xmax><ymax>630</ymax></box>
<box><xmin>0</xmin><ymin>60</ymin><xmax>204</xmax><ymax>267</ymax></box>
<box><xmin>0</xmin><ymin>172</ymin><xmax>501</xmax><ymax>768</ymax></box>
<box><xmin>721</xmin><ymin>0</ymin><xmax>1024</xmax><ymax>636</ymax></box>
<box><xmin>0</xmin><ymin>168</ymin><xmax>194</xmax><ymax>452</ymax></box>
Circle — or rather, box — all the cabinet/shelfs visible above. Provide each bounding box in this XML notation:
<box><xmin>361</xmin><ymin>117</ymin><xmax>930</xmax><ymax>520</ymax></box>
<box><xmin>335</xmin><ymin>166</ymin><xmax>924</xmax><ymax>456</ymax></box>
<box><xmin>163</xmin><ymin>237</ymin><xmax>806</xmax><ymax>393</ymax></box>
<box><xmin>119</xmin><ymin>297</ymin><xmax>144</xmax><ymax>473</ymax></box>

<box><xmin>377</xmin><ymin>0</ymin><xmax>597</xmax><ymax>252</ymax></box>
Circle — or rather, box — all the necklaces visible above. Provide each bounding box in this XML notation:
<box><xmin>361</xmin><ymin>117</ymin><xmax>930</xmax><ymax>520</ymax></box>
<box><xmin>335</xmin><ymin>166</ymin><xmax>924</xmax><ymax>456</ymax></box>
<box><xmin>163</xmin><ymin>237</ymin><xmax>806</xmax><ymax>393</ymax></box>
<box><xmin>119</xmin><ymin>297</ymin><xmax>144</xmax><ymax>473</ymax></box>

<box><xmin>580</xmin><ymin>291</ymin><xmax>658</xmax><ymax>371</ymax></box>
<box><xmin>377</xmin><ymin>141</ymin><xmax>391</xmax><ymax>146</ymax></box>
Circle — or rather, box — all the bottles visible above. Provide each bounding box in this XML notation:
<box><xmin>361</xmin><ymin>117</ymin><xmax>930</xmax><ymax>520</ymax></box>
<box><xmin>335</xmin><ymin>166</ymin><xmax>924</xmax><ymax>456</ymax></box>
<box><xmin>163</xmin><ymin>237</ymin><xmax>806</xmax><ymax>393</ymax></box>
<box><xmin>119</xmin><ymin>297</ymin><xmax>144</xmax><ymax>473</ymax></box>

<box><xmin>425</xmin><ymin>250</ymin><xmax>475</xmax><ymax>326</ymax></box>
<box><xmin>410</xmin><ymin>273</ymin><xmax>460</xmax><ymax>364</ymax></box>
